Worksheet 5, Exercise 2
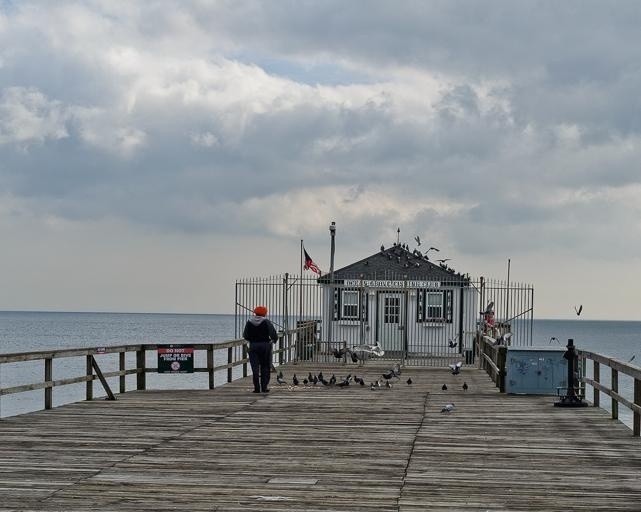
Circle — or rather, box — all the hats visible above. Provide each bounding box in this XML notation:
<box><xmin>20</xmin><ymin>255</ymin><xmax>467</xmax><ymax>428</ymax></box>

<box><xmin>254</xmin><ymin>306</ymin><xmax>267</xmax><ymax>316</ymax></box>
<box><xmin>485</xmin><ymin>310</ymin><xmax>494</xmax><ymax>315</ymax></box>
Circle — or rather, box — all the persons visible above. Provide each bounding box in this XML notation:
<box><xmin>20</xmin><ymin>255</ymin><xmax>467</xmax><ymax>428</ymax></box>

<box><xmin>242</xmin><ymin>305</ymin><xmax>279</xmax><ymax>394</ymax></box>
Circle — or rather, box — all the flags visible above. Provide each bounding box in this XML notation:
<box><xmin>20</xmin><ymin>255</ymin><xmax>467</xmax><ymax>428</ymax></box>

<box><xmin>302</xmin><ymin>244</ymin><xmax>322</xmax><ymax>274</ymax></box>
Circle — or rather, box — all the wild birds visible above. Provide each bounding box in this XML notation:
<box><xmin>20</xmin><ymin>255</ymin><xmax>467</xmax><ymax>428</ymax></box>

<box><xmin>442</xmin><ymin>384</ymin><xmax>448</xmax><ymax>392</ymax></box>
<box><xmin>407</xmin><ymin>377</ymin><xmax>413</xmax><ymax>386</ymax></box>
<box><xmin>363</xmin><ymin>235</ymin><xmax>471</xmax><ymax>280</ymax></box>
<box><xmin>446</xmin><ymin>335</ymin><xmax>458</xmax><ymax>348</ymax></box>
<box><xmin>575</xmin><ymin>304</ymin><xmax>583</xmax><ymax>315</ymax></box>
<box><xmin>448</xmin><ymin>361</ymin><xmax>464</xmax><ymax>375</ymax></box>
<box><xmin>549</xmin><ymin>337</ymin><xmax>563</xmax><ymax>346</ymax></box>
<box><xmin>462</xmin><ymin>382</ymin><xmax>468</xmax><ymax>391</ymax></box>
<box><xmin>276</xmin><ymin>340</ymin><xmax>403</xmax><ymax>393</ymax></box>
<box><xmin>440</xmin><ymin>402</ymin><xmax>455</xmax><ymax>414</ymax></box>
<box><xmin>496</xmin><ymin>331</ymin><xmax>513</xmax><ymax>345</ymax></box>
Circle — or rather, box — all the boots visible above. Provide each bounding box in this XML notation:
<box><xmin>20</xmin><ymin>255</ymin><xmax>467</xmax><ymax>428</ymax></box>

<box><xmin>253</xmin><ymin>379</ymin><xmax>270</xmax><ymax>393</ymax></box>
